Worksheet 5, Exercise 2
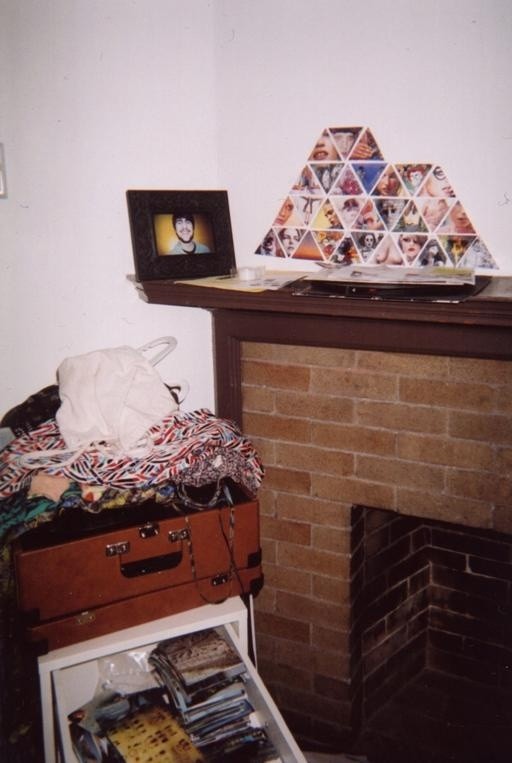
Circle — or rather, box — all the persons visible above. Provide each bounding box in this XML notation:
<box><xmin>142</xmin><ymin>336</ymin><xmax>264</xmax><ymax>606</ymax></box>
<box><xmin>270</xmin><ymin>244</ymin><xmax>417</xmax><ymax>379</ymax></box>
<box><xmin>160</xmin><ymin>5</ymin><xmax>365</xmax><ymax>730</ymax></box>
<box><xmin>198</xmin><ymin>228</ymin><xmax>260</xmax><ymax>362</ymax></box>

<box><xmin>256</xmin><ymin>128</ymin><xmax>473</xmax><ymax>267</ymax></box>
<box><xmin>166</xmin><ymin>211</ymin><xmax>210</xmax><ymax>255</ymax></box>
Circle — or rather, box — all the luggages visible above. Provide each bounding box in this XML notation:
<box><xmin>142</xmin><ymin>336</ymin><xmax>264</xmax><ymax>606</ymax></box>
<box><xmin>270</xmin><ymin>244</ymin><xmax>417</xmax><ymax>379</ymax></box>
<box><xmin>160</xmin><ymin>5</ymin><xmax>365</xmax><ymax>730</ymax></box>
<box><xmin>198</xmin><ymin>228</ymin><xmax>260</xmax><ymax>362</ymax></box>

<box><xmin>14</xmin><ymin>477</ymin><xmax>263</xmax><ymax>630</ymax></box>
<box><xmin>27</xmin><ymin>568</ymin><xmax>263</xmax><ymax>661</ymax></box>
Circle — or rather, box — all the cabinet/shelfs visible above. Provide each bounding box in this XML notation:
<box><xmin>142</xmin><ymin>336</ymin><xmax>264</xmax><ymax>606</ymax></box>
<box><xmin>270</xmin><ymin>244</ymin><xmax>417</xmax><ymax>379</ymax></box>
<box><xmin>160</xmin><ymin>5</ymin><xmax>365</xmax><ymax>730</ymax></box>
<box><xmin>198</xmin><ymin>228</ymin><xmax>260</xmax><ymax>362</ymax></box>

<box><xmin>37</xmin><ymin>595</ymin><xmax>307</xmax><ymax>763</ymax></box>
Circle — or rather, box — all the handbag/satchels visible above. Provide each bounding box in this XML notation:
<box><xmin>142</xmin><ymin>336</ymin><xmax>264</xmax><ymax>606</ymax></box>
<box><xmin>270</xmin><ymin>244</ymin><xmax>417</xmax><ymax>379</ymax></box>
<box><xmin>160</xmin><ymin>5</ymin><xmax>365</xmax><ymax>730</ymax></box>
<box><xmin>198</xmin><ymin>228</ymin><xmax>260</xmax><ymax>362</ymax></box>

<box><xmin>54</xmin><ymin>337</ymin><xmax>187</xmax><ymax>450</ymax></box>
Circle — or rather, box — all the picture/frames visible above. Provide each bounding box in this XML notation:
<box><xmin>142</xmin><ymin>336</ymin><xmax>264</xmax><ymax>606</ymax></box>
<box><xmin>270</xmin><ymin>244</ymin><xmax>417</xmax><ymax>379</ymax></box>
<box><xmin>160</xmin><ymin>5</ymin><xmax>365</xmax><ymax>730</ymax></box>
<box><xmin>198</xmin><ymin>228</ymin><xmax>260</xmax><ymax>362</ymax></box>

<box><xmin>127</xmin><ymin>189</ymin><xmax>238</xmax><ymax>284</ymax></box>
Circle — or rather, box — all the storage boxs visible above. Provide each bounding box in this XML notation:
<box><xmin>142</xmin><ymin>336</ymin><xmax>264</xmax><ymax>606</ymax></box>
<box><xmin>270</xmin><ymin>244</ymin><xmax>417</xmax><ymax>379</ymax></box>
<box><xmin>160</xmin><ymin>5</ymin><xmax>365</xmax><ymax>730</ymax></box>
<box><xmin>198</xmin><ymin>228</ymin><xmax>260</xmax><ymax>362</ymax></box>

<box><xmin>12</xmin><ymin>483</ymin><xmax>266</xmax><ymax>657</ymax></box>
